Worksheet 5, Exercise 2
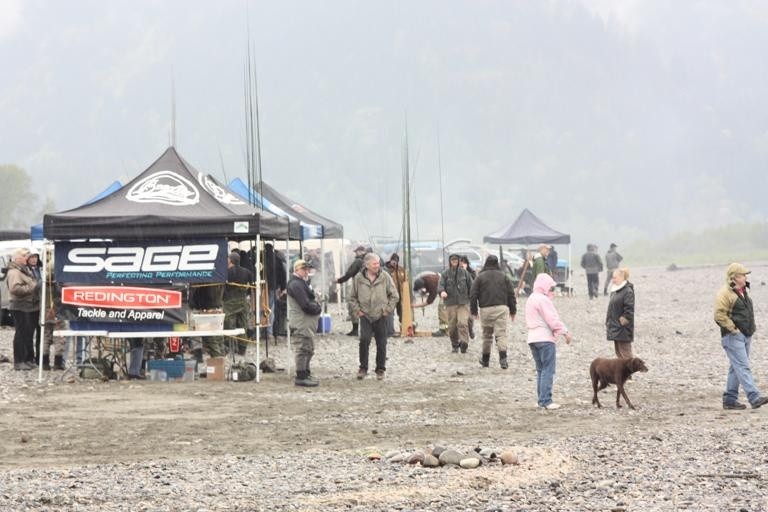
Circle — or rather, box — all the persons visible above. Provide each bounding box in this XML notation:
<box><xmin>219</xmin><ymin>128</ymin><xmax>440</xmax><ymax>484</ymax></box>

<box><xmin>438</xmin><ymin>254</ymin><xmax>474</xmax><ymax>352</ymax></box>
<box><xmin>606</xmin><ymin>268</ymin><xmax>635</xmax><ymax>379</ymax></box>
<box><xmin>286</xmin><ymin>261</ymin><xmax>321</xmax><ymax>386</ymax></box>
<box><xmin>253</xmin><ymin>244</ymin><xmax>285</xmax><ymax>346</ymax></box>
<box><xmin>532</xmin><ymin>245</ymin><xmax>552</xmax><ymax>279</ymax></box>
<box><xmin>129</xmin><ymin>337</ymin><xmax>144</xmax><ymax>379</ymax></box>
<box><xmin>349</xmin><ymin>252</ymin><xmax>399</xmax><ymax>379</ymax></box>
<box><xmin>7</xmin><ymin>250</ymin><xmax>38</xmax><ymax>371</ymax></box>
<box><xmin>367</xmin><ymin>249</ymin><xmax>388</xmax><ymax>275</ymax></box>
<box><xmin>379</xmin><ymin>254</ymin><xmax>405</xmax><ymax>328</ymax></box>
<box><xmin>526</xmin><ymin>274</ymin><xmax>571</xmax><ymax>410</ymax></box>
<box><xmin>25</xmin><ymin>250</ymin><xmax>42</xmax><ymax>368</ymax></box>
<box><xmin>604</xmin><ymin>244</ymin><xmax>622</xmax><ymax>294</ymax></box>
<box><xmin>35</xmin><ymin>262</ymin><xmax>69</xmax><ymax>370</ymax></box>
<box><xmin>714</xmin><ymin>263</ymin><xmax>767</xmax><ymax>409</ymax></box>
<box><xmin>582</xmin><ymin>245</ymin><xmax>602</xmax><ymax>300</ymax></box>
<box><xmin>333</xmin><ymin>247</ymin><xmax>367</xmax><ymax>337</ymax></box>
<box><xmin>459</xmin><ymin>257</ymin><xmax>477</xmax><ymax>339</ymax></box>
<box><xmin>224</xmin><ymin>254</ymin><xmax>253</xmax><ymax>356</ymax></box>
<box><xmin>231</xmin><ymin>245</ymin><xmax>259</xmax><ymax>268</ymax></box>
<box><xmin>411</xmin><ymin>274</ymin><xmax>448</xmax><ymax>337</ymax></box>
<box><xmin>470</xmin><ymin>254</ymin><xmax>517</xmax><ymax>369</ymax></box>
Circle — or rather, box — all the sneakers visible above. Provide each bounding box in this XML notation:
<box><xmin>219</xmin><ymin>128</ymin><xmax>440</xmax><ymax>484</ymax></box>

<box><xmin>356</xmin><ymin>368</ymin><xmax>367</xmax><ymax>380</ymax></box>
<box><xmin>593</xmin><ymin>290</ymin><xmax>598</xmax><ymax>298</ymax></box>
<box><xmin>723</xmin><ymin>402</ymin><xmax>746</xmax><ymax>411</ymax></box>
<box><xmin>542</xmin><ymin>402</ymin><xmax>563</xmax><ymax>410</ymax></box>
<box><xmin>376</xmin><ymin>372</ymin><xmax>385</xmax><ymax>381</ymax></box>
<box><xmin>25</xmin><ymin>362</ymin><xmax>37</xmax><ymax>368</ymax></box>
<box><xmin>14</xmin><ymin>362</ymin><xmax>31</xmax><ymax>371</ymax></box>
<box><xmin>432</xmin><ymin>329</ymin><xmax>445</xmax><ymax>337</ymax></box>
<box><xmin>469</xmin><ymin>331</ymin><xmax>475</xmax><ymax>339</ymax></box>
<box><xmin>296</xmin><ymin>378</ymin><xmax>319</xmax><ymax>387</ymax></box>
<box><xmin>752</xmin><ymin>397</ymin><xmax>768</xmax><ymax>409</ymax></box>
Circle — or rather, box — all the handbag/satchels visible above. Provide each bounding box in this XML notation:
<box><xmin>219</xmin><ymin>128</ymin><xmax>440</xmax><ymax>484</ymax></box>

<box><xmin>228</xmin><ymin>364</ymin><xmax>258</xmax><ymax>384</ymax></box>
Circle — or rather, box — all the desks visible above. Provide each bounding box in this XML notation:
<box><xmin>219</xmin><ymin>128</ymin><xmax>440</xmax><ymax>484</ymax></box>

<box><xmin>107</xmin><ymin>328</ymin><xmax>245</xmax><ymax>382</ymax></box>
<box><xmin>53</xmin><ymin>330</ymin><xmax>128</xmax><ymax>382</ymax></box>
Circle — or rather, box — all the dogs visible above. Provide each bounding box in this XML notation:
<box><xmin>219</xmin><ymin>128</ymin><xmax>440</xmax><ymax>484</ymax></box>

<box><xmin>590</xmin><ymin>357</ymin><xmax>649</xmax><ymax>412</ymax></box>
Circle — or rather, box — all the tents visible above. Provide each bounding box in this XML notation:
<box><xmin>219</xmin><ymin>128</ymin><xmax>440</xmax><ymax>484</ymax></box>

<box><xmin>254</xmin><ymin>181</ymin><xmax>345</xmax><ymax>297</ymax></box>
<box><xmin>483</xmin><ymin>210</ymin><xmax>570</xmax><ymax>271</ymax></box>
<box><xmin>227</xmin><ymin>178</ymin><xmax>325</xmax><ymax>335</ymax></box>
<box><xmin>31</xmin><ymin>182</ymin><xmax>122</xmax><ymax>241</ymax></box>
<box><xmin>223</xmin><ymin>176</ymin><xmax>344</xmax><ymax>338</ymax></box>
<box><xmin>39</xmin><ymin>147</ymin><xmax>290</xmax><ymax>385</ymax></box>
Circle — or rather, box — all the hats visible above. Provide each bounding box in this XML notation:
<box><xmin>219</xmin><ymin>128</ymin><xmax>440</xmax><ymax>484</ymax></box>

<box><xmin>294</xmin><ymin>259</ymin><xmax>313</xmax><ymax>270</ymax></box>
<box><xmin>10</xmin><ymin>248</ymin><xmax>30</xmax><ymax>258</ymax></box>
<box><xmin>354</xmin><ymin>245</ymin><xmax>366</xmax><ymax>251</ymax></box>
<box><xmin>390</xmin><ymin>254</ymin><xmax>400</xmax><ymax>261</ymax></box>
<box><xmin>727</xmin><ymin>262</ymin><xmax>752</xmax><ymax>276</ymax></box>
<box><xmin>413</xmin><ymin>279</ymin><xmax>425</xmax><ymax>291</ymax></box>
<box><xmin>536</xmin><ymin>244</ymin><xmax>551</xmax><ymax>251</ymax></box>
<box><xmin>610</xmin><ymin>244</ymin><xmax>617</xmax><ymax>247</ymax></box>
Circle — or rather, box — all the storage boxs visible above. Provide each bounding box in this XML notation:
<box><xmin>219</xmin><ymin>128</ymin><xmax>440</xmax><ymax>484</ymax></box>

<box><xmin>150</xmin><ymin>359</ymin><xmax>197</xmax><ymax>381</ymax></box>
<box><xmin>206</xmin><ymin>358</ymin><xmax>223</xmax><ymax>380</ymax></box>
<box><xmin>189</xmin><ymin>309</ymin><xmax>225</xmax><ymax>329</ymax></box>
<box><xmin>556</xmin><ymin>259</ymin><xmax>569</xmax><ymax>278</ymax></box>
<box><xmin>317</xmin><ymin>314</ymin><xmax>331</xmax><ymax>333</ymax></box>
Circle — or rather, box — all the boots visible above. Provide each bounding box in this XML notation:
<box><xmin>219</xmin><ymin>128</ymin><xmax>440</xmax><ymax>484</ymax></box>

<box><xmin>347</xmin><ymin>323</ymin><xmax>359</xmax><ymax>336</ymax></box>
<box><xmin>499</xmin><ymin>351</ymin><xmax>508</xmax><ymax>369</ymax></box>
<box><xmin>40</xmin><ymin>353</ymin><xmax>63</xmax><ymax>374</ymax></box>
<box><xmin>479</xmin><ymin>354</ymin><xmax>490</xmax><ymax>368</ymax></box>
<box><xmin>128</xmin><ymin>346</ymin><xmax>146</xmax><ymax>378</ymax></box>
<box><xmin>603</xmin><ymin>288</ymin><xmax>609</xmax><ymax>295</ymax></box>
